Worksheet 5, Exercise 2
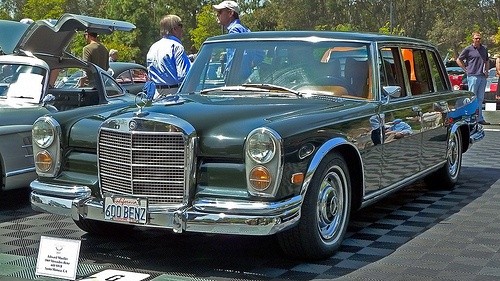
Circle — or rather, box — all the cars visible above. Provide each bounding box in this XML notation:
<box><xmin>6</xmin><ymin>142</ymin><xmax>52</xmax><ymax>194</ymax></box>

<box><xmin>29</xmin><ymin>30</ymin><xmax>485</xmax><ymax>259</ymax></box>
<box><xmin>0</xmin><ymin>12</ymin><xmax>148</xmax><ymax>193</ymax></box>
<box><xmin>460</xmin><ymin>67</ymin><xmax>500</xmax><ymax>109</ymax></box>
<box><xmin>58</xmin><ymin>61</ymin><xmax>150</xmax><ymax>98</ymax></box>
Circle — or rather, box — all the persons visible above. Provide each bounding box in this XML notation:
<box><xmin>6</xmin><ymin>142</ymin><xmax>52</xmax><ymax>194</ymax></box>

<box><xmin>77</xmin><ymin>30</ymin><xmax>109</xmax><ymax>87</ymax></box>
<box><xmin>439</xmin><ymin>100</ymin><xmax>450</xmax><ymax>126</ymax></box>
<box><xmin>456</xmin><ymin>32</ymin><xmax>490</xmax><ymax>125</ymax></box>
<box><xmin>145</xmin><ymin>14</ymin><xmax>191</xmax><ymax>99</ymax></box>
<box><xmin>212</xmin><ymin>0</ymin><xmax>266</xmax><ymax>86</ymax></box>
<box><xmin>109</xmin><ymin>49</ymin><xmax>120</xmax><ymax>62</ymax></box>
<box><xmin>370</xmin><ymin>112</ymin><xmax>386</xmax><ymax>145</ymax></box>
<box><xmin>385</xmin><ymin>119</ymin><xmax>413</xmax><ymax>139</ymax></box>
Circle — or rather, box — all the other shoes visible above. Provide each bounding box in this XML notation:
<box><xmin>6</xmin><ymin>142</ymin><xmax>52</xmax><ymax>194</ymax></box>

<box><xmin>479</xmin><ymin>120</ymin><xmax>490</xmax><ymax>125</ymax></box>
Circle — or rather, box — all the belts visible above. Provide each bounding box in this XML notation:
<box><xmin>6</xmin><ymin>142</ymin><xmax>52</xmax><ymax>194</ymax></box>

<box><xmin>155</xmin><ymin>84</ymin><xmax>180</xmax><ymax>89</ymax></box>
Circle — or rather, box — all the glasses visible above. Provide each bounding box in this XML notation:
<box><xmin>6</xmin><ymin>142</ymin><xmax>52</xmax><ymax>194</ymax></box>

<box><xmin>178</xmin><ymin>23</ymin><xmax>183</xmax><ymax>28</ymax></box>
<box><xmin>473</xmin><ymin>38</ymin><xmax>482</xmax><ymax>40</ymax></box>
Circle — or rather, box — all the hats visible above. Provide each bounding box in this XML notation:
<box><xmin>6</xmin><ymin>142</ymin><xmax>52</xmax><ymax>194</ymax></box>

<box><xmin>212</xmin><ymin>0</ymin><xmax>240</xmax><ymax>14</ymax></box>
